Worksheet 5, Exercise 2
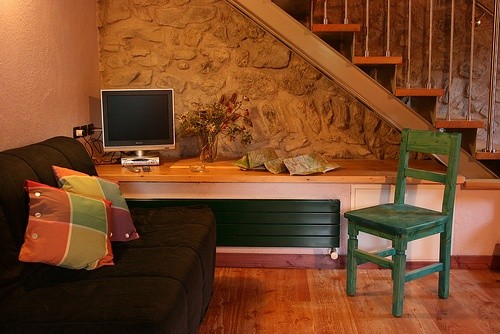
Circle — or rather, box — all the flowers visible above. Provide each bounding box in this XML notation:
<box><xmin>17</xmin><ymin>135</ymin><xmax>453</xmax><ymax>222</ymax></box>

<box><xmin>174</xmin><ymin>93</ymin><xmax>253</xmax><ymax>145</ymax></box>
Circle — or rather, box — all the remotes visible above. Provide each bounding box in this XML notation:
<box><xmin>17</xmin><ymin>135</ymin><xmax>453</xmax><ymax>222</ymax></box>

<box><xmin>128</xmin><ymin>166</ymin><xmax>139</xmax><ymax>173</ymax></box>
<box><xmin>142</xmin><ymin>166</ymin><xmax>150</xmax><ymax>172</ymax></box>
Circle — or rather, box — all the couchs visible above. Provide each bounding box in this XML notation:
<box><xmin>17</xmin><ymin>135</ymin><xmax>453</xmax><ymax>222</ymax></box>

<box><xmin>0</xmin><ymin>136</ymin><xmax>216</xmax><ymax>334</ymax></box>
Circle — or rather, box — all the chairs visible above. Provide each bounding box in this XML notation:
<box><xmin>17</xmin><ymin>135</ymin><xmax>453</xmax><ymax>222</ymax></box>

<box><xmin>343</xmin><ymin>128</ymin><xmax>462</xmax><ymax>318</ymax></box>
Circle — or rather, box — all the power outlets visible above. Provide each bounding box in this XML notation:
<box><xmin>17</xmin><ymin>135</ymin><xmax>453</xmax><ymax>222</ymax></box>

<box><xmin>73</xmin><ymin>123</ymin><xmax>94</xmax><ymax>140</ymax></box>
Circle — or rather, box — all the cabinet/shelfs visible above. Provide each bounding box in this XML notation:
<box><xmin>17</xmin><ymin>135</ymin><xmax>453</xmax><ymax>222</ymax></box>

<box><xmin>92</xmin><ymin>156</ymin><xmax>500</xmax><ymax>271</ymax></box>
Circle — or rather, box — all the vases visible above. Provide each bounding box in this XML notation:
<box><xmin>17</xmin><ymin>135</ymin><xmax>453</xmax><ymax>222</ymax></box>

<box><xmin>198</xmin><ymin>135</ymin><xmax>218</xmax><ymax>163</ymax></box>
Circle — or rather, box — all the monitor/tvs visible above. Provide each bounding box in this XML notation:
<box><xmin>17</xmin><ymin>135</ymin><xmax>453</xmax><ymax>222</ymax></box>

<box><xmin>101</xmin><ymin>89</ymin><xmax>175</xmax><ymax>151</ymax></box>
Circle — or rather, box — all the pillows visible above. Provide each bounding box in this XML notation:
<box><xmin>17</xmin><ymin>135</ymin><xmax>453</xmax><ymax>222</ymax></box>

<box><xmin>18</xmin><ymin>180</ymin><xmax>115</xmax><ymax>271</ymax></box>
<box><xmin>52</xmin><ymin>165</ymin><xmax>139</xmax><ymax>241</ymax></box>
